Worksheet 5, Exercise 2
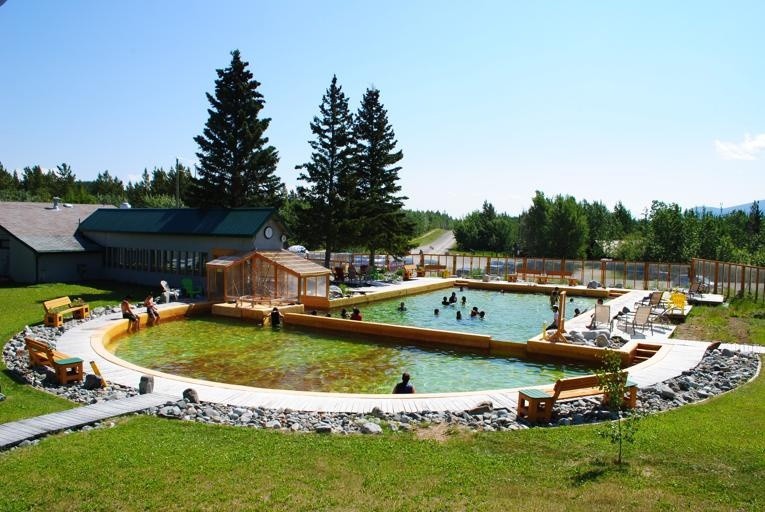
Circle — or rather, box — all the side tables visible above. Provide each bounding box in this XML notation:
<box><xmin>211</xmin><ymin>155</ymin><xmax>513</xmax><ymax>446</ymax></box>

<box><xmin>537</xmin><ymin>276</ymin><xmax>548</xmax><ymax>284</ymax></box>
<box><xmin>568</xmin><ymin>278</ymin><xmax>576</xmax><ymax>286</ymax></box>
<box><xmin>623</xmin><ymin>380</ymin><xmax>639</xmax><ymax>408</ymax></box>
<box><xmin>507</xmin><ymin>274</ymin><xmax>518</xmax><ymax>282</ymax></box>
<box><xmin>73</xmin><ymin>303</ymin><xmax>89</xmax><ymax>319</ymax></box>
<box><xmin>43</xmin><ymin>312</ymin><xmax>63</xmax><ymax>327</ymax></box>
<box><xmin>54</xmin><ymin>357</ymin><xmax>84</xmax><ymax>385</ymax></box>
<box><xmin>517</xmin><ymin>387</ymin><xmax>552</xmax><ymax>425</ymax></box>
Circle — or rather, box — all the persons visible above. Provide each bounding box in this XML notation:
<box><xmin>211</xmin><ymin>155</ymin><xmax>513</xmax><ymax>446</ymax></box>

<box><xmin>143</xmin><ymin>291</ymin><xmax>160</xmax><ymax>327</ymax></box>
<box><xmin>433</xmin><ymin>286</ymin><xmax>484</xmax><ymax>320</ymax></box>
<box><xmin>121</xmin><ymin>294</ymin><xmax>141</xmax><ymax>332</ymax></box>
<box><xmin>392</xmin><ymin>372</ymin><xmax>416</xmax><ymax>394</ymax></box>
<box><xmin>268</xmin><ymin>307</ymin><xmax>283</xmax><ymax>329</ymax></box>
<box><xmin>311</xmin><ymin>306</ymin><xmax>362</xmax><ymax>321</ymax></box>
<box><xmin>545</xmin><ymin>287</ymin><xmax>587</xmax><ymax>332</ymax></box>
<box><xmin>397</xmin><ymin>302</ymin><xmax>406</xmax><ymax>311</ymax></box>
<box><xmin>586</xmin><ymin>299</ymin><xmax>603</xmax><ymax>328</ymax></box>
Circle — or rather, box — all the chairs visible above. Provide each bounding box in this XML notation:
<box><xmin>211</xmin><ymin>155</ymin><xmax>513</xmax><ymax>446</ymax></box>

<box><xmin>593</xmin><ymin>302</ymin><xmax>615</xmax><ymax>332</ymax></box>
<box><xmin>332</xmin><ymin>264</ymin><xmax>368</xmax><ymax>284</ymax></box>
<box><xmin>161</xmin><ymin>277</ymin><xmax>203</xmax><ymax>302</ymax></box>
<box><xmin>685</xmin><ymin>283</ymin><xmax>705</xmax><ymax>298</ymax></box>
<box><xmin>616</xmin><ymin>290</ymin><xmax>689</xmax><ymax>338</ymax></box>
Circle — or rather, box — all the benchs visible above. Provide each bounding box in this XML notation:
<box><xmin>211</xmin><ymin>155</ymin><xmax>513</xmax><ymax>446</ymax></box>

<box><xmin>42</xmin><ymin>295</ymin><xmax>85</xmax><ymax>327</ymax></box>
<box><xmin>24</xmin><ymin>337</ymin><xmax>71</xmax><ymax>382</ymax></box>
<box><xmin>543</xmin><ymin>369</ymin><xmax>629</xmax><ymax>426</ymax></box>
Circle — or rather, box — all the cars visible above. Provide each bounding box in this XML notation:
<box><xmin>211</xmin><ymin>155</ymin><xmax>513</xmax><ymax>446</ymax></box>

<box><xmin>416</xmin><ymin>260</ymin><xmax>437</xmax><ymax>269</ymax></box>
<box><xmin>628</xmin><ymin>266</ymin><xmax>715</xmax><ymax>289</ymax></box>
<box><xmin>489</xmin><ymin>260</ymin><xmax>574</xmax><ymax>274</ymax></box>
<box><xmin>457</xmin><ymin>267</ymin><xmax>470</xmax><ymax>277</ymax></box>
<box><xmin>353</xmin><ymin>255</ymin><xmax>413</xmax><ymax>271</ymax></box>
